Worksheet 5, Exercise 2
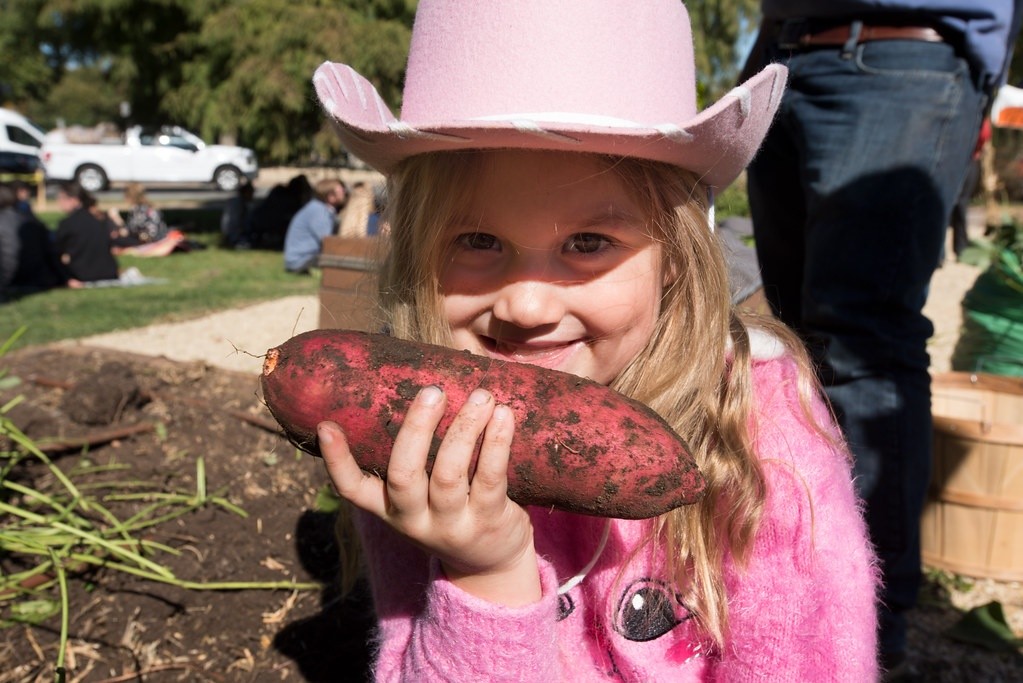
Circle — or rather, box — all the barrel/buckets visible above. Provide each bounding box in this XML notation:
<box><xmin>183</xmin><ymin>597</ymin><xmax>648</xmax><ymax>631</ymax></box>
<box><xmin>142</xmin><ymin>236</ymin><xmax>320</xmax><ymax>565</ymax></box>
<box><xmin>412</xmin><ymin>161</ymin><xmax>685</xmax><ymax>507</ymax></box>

<box><xmin>319</xmin><ymin>236</ymin><xmax>391</xmax><ymax>333</ymax></box>
<box><xmin>920</xmin><ymin>371</ymin><xmax>1023</xmax><ymax>583</ymax></box>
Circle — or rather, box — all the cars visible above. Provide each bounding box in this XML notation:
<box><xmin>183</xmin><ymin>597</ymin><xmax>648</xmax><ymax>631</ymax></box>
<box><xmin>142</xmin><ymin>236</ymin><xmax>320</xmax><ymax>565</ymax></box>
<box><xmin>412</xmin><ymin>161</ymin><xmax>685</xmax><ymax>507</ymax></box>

<box><xmin>1</xmin><ymin>109</ymin><xmax>44</xmax><ymax>178</ymax></box>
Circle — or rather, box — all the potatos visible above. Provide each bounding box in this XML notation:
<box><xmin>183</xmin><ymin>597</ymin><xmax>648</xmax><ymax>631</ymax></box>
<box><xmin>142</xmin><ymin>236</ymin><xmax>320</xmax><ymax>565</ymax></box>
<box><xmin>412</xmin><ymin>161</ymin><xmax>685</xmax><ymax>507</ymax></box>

<box><xmin>259</xmin><ymin>330</ymin><xmax>706</xmax><ymax>519</ymax></box>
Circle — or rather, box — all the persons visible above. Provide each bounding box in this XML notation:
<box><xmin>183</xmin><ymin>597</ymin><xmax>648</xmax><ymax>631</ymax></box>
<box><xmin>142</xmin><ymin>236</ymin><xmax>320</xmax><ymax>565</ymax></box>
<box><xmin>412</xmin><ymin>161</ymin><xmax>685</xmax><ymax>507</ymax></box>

<box><xmin>312</xmin><ymin>0</ymin><xmax>883</xmax><ymax>683</ymax></box>
<box><xmin>221</xmin><ymin>174</ymin><xmax>379</xmax><ymax>273</ymax></box>
<box><xmin>0</xmin><ymin>180</ymin><xmax>164</xmax><ymax>302</ymax></box>
<box><xmin>737</xmin><ymin>0</ymin><xmax>1023</xmax><ymax>668</ymax></box>
<box><xmin>937</xmin><ymin>114</ymin><xmax>991</xmax><ymax>267</ymax></box>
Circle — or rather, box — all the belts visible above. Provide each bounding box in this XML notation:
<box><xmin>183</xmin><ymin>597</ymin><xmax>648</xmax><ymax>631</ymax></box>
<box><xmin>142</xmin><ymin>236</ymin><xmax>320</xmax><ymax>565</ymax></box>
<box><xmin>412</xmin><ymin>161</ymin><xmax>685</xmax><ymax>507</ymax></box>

<box><xmin>759</xmin><ymin>15</ymin><xmax>943</xmax><ymax>47</ymax></box>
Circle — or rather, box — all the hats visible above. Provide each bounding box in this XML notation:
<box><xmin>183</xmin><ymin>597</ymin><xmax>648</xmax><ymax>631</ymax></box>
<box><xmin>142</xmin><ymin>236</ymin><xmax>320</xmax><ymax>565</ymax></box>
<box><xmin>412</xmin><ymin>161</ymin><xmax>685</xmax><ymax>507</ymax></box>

<box><xmin>312</xmin><ymin>1</ymin><xmax>789</xmax><ymax>205</ymax></box>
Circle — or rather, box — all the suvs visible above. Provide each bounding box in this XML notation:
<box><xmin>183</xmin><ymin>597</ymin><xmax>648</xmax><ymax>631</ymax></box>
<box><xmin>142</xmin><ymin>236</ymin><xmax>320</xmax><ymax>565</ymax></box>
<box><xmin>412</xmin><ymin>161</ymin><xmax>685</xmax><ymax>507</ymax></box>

<box><xmin>40</xmin><ymin>124</ymin><xmax>260</xmax><ymax>192</ymax></box>
